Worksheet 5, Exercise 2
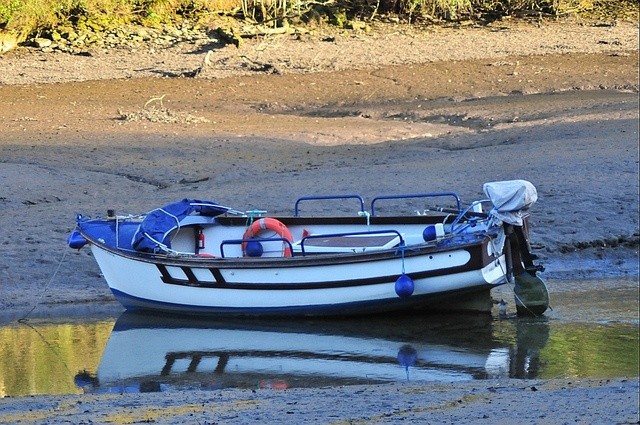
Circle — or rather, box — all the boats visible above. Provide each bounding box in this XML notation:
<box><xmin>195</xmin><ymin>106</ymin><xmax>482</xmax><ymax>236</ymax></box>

<box><xmin>67</xmin><ymin>181</ymin><xmax>549</xmax><ymax>316</ymax></box>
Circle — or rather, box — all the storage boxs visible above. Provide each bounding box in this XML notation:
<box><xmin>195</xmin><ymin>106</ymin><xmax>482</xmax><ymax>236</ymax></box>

<box><xmin>290</xmin><ymin>233</ymin><xmax>406</xmax><ymax>257</ymax></box>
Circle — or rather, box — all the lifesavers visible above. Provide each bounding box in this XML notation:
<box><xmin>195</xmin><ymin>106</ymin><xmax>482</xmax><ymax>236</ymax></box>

<box><xmin>242</xmin><ymin>218</ymin><xmax>293</xmax><ymax>257</ymax></box>
